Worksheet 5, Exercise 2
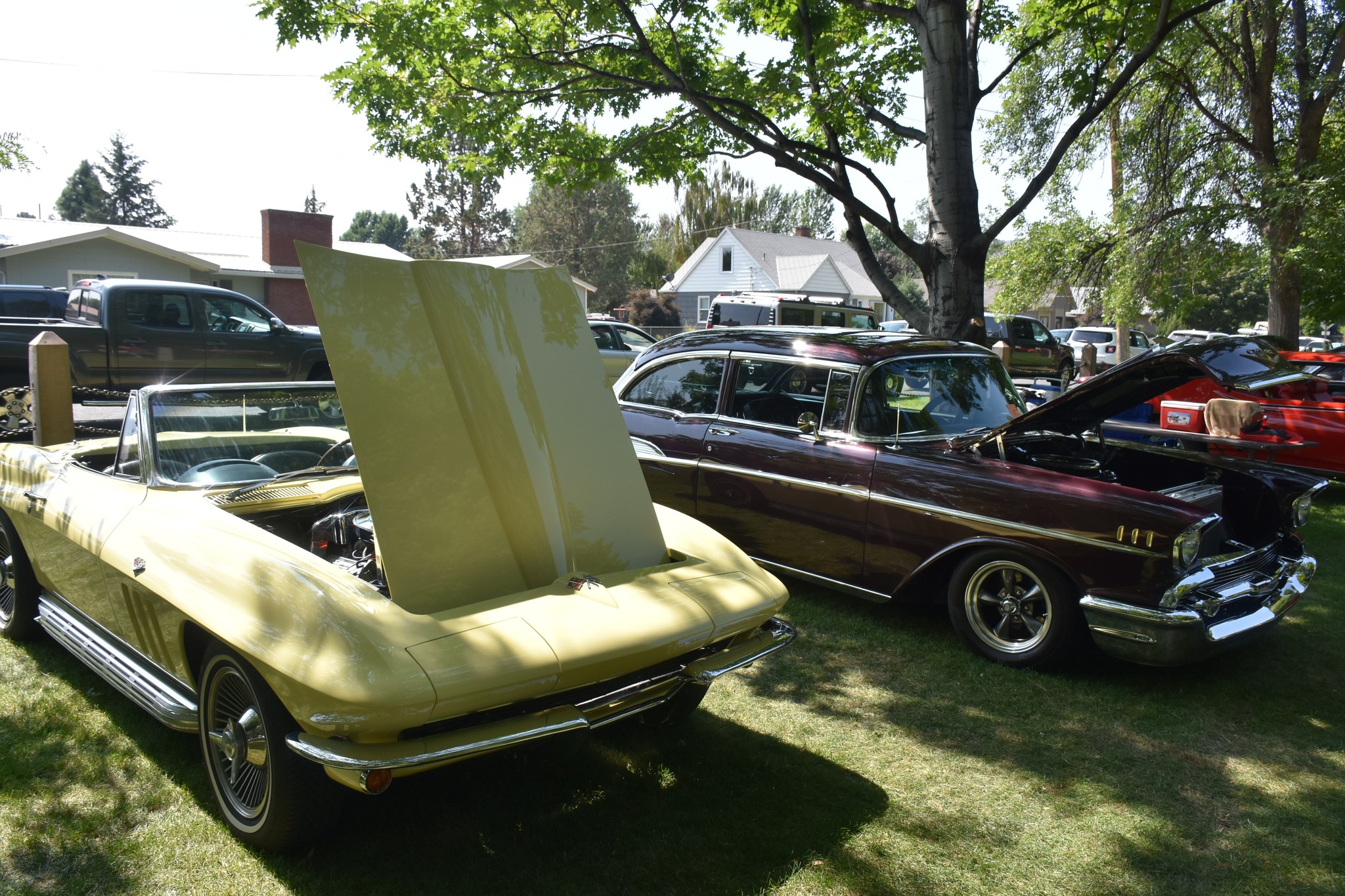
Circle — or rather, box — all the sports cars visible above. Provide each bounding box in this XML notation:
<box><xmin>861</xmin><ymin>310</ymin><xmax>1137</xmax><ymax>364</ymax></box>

<box><xmin>1079</xmin><ymin>349</ymin><xmax>1345</xmax><ymax>483</ymax></box>
<box><xmin>0</xmin><ymin>238</ymin><xmax>798</xmax><ymax>854</ymax></box>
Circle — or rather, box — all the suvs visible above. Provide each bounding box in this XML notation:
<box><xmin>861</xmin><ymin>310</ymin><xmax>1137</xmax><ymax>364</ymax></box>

<box><xmin>705</xmin><ymin>292</ymin><xmax>886</xmax><ymax>395</ymax></box>
<box><xmin>0</xmin><ymin>283</ymin><xmax>70</xmax><ymax>321</ymax></box>
<box><xmin>1066</xmin><ymin>325</ymin><xmax>1151</xmax><ymax>380</ymax></box>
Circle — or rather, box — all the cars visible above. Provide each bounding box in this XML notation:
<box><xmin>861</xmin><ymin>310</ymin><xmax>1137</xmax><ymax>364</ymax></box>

<box><xmin>610</xmin><ymin>328</ymin><xmax>1331</xmax><ymax>671</ymax></box>
<box><xmin>586</xmin><ymin>315</ymin><xmax>683</xmax><ymax>389</ymax></box>
<box><xmin>1167</xmin><ymin>320</ymin><xmax>1345</xmax><ymax>397</ymax></box>
<box><xmin>1041</xmin><ymin>328</ymin><xmax>1074</xmax><ymax>343</ymax></box>
<box><xmin>879</xmin><ymin>320</ymin><xmax>908</xmax><ymax>332</ymax></box>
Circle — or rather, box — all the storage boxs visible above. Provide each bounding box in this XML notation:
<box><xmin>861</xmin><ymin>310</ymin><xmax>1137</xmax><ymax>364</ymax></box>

<box><xmin>1160</xmin><ymin>400</ymin><xmax>1208</xmax><ymax>432</ymax></box>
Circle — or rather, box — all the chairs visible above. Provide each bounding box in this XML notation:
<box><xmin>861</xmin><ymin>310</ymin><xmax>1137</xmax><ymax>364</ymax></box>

<box><xmin>101</xmin><ymin>458</ymin><xmax>194</xmax><ymax>479</ymax></box>
<box><xmin>1093</xmin><ymin>404</ymin><xmax>1180</xmax><ymax>448</ymax></box>
<box><xmin>251</xmin><ymin>450</ymin><xmax>330</xmax><ymax>471</ymax></box>
<box><xmin>141</xmin><ymin>302</ymin><xmax>182</xmax><ymax>328</ymax></box>
<box><xmin>827</xmin><ymin>392</ymin><xmax>891</xmax><ymax>436</ymax></box>
<box><xmin>742</xmin><ymin>394</ymin><xmax>808</xmax><ymax>426</ymax></box>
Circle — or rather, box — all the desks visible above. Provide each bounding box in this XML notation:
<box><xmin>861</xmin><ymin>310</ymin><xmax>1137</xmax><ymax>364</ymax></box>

<box><xmin>1097</xmin><ymin>418</ymin><xmax>1318</xmax><ymax>466</ymax></box>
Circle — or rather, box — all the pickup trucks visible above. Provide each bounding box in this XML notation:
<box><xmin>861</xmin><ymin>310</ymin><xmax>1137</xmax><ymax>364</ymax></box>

<box><xmin>0</xmin><ymin>274</ymin><xmax>346</xmax><ymax>443</ymax></box>
<box><xmin>898</xmin><ymin>311</ymin><xmax>1076</xmax><ymax>388</ymax></box>
